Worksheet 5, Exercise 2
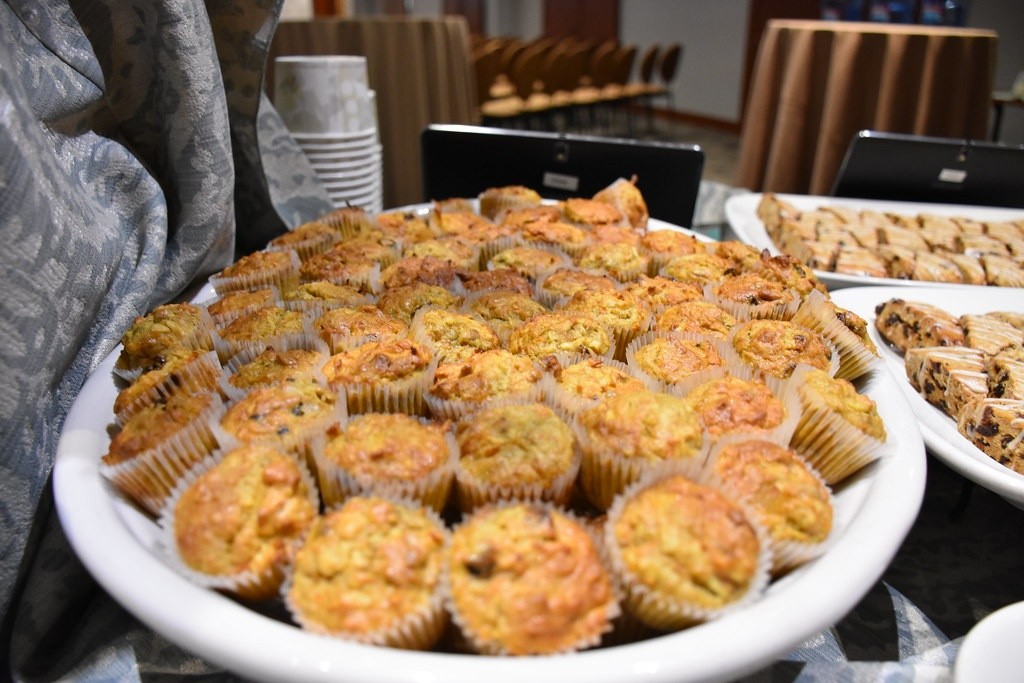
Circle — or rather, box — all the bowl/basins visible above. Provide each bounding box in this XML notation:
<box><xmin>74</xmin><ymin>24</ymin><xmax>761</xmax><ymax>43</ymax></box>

<box><xmin>272</xmin><ymin>53</ymin><xmax>383</xmax><ymax>216</ymax></box>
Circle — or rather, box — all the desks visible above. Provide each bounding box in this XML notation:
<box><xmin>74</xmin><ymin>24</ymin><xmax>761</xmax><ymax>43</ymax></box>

<box><xmin>263</xmin><ymin>16</ymin><xmax>477</xmax><ymax>206</ymax></box>
<box><xmin>734</xmin><ymin>17</ymin><xmax>999</xmax><ymax>205</ymax></box>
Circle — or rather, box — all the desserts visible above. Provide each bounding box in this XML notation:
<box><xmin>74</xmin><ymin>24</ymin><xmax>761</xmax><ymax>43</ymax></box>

<box><xmin>102</xmin><ymin>178</ymin><xmax>885</xmax><ymax>657</ymax></box>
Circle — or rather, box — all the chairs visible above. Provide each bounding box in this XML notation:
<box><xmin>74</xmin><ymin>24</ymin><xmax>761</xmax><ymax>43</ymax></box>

<box><xmin>471</xmin><ymin>27</ymin><xmax>685</xmax><ymax>141</ymax></box>
<box><xmin>991</xmin><ymin>68</ymin><xmax>1024</xmax><ymax>145</ymax></box>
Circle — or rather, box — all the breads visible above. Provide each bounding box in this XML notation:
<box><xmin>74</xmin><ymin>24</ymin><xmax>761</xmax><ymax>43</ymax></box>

<box><xmin>757</xmin><ymin>191</ymin><xmax>1024</xmax><ymax>476</ymax></box>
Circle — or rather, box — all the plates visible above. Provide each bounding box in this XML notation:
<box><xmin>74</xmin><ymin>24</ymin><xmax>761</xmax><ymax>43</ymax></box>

<box><xmin>725</xmin><ymin>191</ymin><xmax>1024</xmax><ymax>291</ymax></box>
<box><xmin>52</xmin><ymin>196</ymin><xmax>928</xmax><ymax>683</ymax></box>
<box><xmin>829</xmin><ymin>285</ymin><xmax>1024</xmax><ymax>509</ymax></box>
